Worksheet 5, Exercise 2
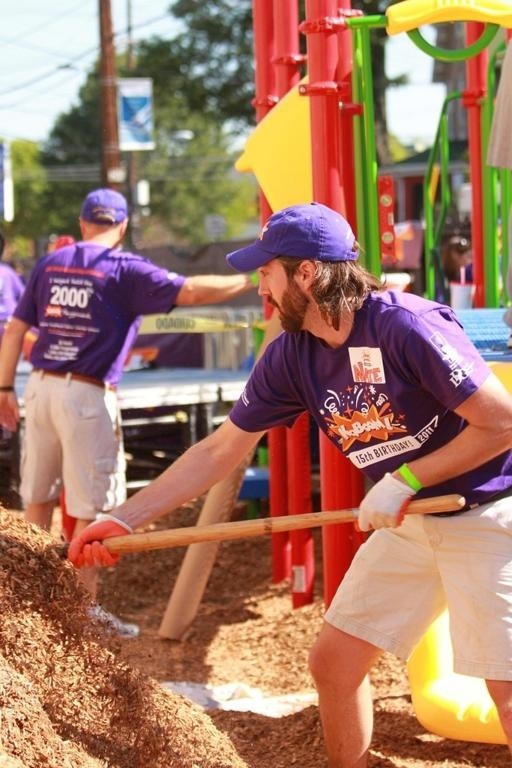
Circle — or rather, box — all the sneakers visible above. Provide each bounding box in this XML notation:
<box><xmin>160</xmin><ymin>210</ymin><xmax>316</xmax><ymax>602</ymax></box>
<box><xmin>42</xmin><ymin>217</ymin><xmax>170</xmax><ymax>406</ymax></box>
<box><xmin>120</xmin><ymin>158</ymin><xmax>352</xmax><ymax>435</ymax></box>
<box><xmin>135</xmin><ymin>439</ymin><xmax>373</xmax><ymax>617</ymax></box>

<box><xmin>88</xmin><ymin>606</ymin><xmax>139</xmax><ymax>640</ymax></box>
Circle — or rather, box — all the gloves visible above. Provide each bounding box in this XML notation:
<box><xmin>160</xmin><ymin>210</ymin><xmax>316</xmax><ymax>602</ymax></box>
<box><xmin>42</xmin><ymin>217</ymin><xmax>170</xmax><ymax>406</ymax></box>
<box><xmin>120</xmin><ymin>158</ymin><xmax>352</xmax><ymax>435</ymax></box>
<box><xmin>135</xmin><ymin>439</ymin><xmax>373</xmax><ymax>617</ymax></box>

<box><xmin>354</xmin><ymin>472</ymin><xmax>416</xmax><ymax>533</ymax></box>
<box><xmin>67</xmin><ymin>514</ymin><xmax>133</xmax><ymax>568</ymax></box>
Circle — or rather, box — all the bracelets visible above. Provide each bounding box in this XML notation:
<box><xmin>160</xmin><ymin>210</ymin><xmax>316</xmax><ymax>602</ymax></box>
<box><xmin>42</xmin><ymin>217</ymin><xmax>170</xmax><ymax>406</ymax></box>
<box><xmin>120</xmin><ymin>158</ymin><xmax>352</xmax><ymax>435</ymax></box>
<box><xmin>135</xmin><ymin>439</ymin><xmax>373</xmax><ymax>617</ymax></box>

<box><xmin>249</xmin><ymin>271</ymin><xmax>261</xmax><ymax>290</ymax></box>
<box><xmin>0</xmin><ymin>385</ymin><xmax>13</xmax><ymax>394</ymax></box>
<box><xmin>397</xmin><ymin>460</ymin><xmax>423</xmax><ymax>492</ymax></box>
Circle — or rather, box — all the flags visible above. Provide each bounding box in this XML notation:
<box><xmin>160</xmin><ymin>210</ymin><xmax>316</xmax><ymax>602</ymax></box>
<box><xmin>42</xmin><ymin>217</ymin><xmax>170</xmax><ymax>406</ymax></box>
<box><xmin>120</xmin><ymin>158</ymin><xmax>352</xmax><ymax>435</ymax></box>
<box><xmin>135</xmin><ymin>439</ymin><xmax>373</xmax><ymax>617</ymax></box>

<box><xmin>116</xmin><ymin>74</ymin><xmax>156</xmax><ymax>153</ymax></box>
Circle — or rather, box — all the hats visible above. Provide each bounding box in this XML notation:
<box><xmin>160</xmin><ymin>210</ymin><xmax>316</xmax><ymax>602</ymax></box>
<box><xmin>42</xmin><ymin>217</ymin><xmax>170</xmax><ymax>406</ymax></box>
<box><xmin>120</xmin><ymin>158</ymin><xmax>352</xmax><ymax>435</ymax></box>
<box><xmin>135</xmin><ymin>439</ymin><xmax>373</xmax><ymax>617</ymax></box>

<box><xmin>82</xmin><ymin>189</ymin><xmax>128</xmax><ymax>225</ymax></box>
<box><xmin>226</xmin><ymin>202</ymin><xmax>357</xmax><ymax>272</ymax></box>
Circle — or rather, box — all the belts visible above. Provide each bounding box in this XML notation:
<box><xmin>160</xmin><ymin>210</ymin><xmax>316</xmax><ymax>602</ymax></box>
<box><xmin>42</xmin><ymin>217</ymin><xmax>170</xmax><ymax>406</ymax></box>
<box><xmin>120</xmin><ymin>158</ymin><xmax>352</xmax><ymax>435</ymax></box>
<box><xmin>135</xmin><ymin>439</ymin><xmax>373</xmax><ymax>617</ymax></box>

<box><xmin>46</xmin><ymin>367</ymin><xmax>104</xmax><ymax>387</ymax></box>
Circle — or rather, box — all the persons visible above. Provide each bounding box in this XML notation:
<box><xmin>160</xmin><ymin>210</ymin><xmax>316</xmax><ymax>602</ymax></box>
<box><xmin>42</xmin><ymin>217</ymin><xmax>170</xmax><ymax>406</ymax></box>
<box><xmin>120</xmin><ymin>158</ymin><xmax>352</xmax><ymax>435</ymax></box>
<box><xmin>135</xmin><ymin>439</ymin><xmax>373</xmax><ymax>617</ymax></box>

<box><xmin>443</xmin><ymin>234</ymin><xmax>473</xmax><ymax>308</ymax></box>
<box><xmin>65</xmin><ymin>203</ymin><xmax>511</xmax><ymax>768</ymax></box>
<box><xmin>0</xmin><ymin>185</ymin><xmax>259</xmax><ymax>640</ymax></box>
<box><xmin>0</xmin><ymin>236</ymin><xmax>31</xmax><ymax>357</ymax></box>
